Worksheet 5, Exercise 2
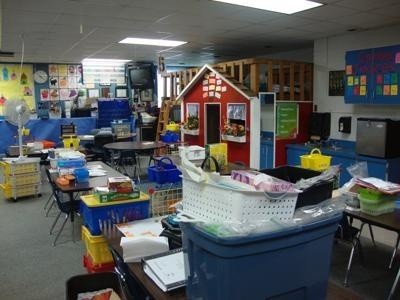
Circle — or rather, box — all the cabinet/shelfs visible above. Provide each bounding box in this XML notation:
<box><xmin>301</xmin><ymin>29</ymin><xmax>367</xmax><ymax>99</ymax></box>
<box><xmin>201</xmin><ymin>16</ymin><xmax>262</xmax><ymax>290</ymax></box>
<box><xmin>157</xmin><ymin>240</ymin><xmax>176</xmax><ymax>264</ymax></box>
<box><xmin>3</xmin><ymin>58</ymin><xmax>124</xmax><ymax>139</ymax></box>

<box><xmin>344</xmin><ymin>44</ymin><xmax>399</xmax><ymax>106</ymax></box>
<box><xmin>332</xmin><ymin>156</ymin><xmax>399</xmax><ymax>189</ymax></box>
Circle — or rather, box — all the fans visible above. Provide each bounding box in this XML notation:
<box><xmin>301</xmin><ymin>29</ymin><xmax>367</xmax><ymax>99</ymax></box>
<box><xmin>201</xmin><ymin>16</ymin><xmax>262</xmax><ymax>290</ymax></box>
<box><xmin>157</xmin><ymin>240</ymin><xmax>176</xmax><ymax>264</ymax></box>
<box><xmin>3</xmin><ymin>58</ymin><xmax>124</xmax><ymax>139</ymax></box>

<box><xmin>3</xmin><ymin>95</ymin><xmax>31</xmax><ymax>163</ymax></box>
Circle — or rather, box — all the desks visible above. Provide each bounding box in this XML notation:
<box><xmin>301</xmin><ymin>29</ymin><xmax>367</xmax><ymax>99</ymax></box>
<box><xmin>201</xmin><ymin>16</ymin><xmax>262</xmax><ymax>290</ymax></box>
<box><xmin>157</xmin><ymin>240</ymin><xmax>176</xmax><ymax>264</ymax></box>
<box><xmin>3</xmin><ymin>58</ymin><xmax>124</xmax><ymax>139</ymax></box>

<box><xmin>54</xmin><ymin>159</ymin><xmax>133</xmax><ymax>243</ymax></box>
<box><xmin>103</xmin><ymin>140</ymin><xmax>168</xmax><ymax>182</ymax></box>
<box><xmin>343</xmin><ymin>202</ymin><xmax>399</xmax><ymax>299</ymax></box>
<box><xmin>108</xmin><ymin>232</ymin><xmax>367</xmax><ymax>299</ymax></box>
<box><xmin>59</xmin><ymin>134</ymin><xmax>95</xmax><ymax>148</ymax></box>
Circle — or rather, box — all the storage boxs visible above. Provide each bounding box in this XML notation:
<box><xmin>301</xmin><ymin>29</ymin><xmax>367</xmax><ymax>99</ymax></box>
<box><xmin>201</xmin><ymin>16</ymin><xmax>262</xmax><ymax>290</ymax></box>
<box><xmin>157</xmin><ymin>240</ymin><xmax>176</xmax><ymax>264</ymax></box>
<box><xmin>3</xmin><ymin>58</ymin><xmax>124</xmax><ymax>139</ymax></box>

<box><xmin>180</xmin><ymin>212</ymin><xmax>342</xmax><ymax>299</ymax></box>
<box><xmin>79</xmin><ymin>192</ymin><xmax>151</xmax><ymax>273</ymax></box>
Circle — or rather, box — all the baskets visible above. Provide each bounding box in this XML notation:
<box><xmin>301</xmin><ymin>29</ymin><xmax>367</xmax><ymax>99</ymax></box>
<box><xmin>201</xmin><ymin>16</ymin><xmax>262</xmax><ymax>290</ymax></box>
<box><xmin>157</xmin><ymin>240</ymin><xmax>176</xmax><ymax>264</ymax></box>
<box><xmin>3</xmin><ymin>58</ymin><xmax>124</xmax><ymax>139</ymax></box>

<box><xmin>0</xmin><ymin>160</ymin><xmax>41</xmax><ymax>198</ymax></box>
<box><xmin>80</xmin><ymin>191</ymin><xmax>149</xmax><ymax>272</ymax></box>
<box><xmin>179</xmin><ymin>172</ymin><xmax>302</xmax><ymax>230</ymax></box>
<box><xmin>299</xmin><ymin>148</ymin><xmax>332</xmax><ymax>172</ymax></box>
<box><xmin>358</xmin><ymin>188</ymin><xmax>396</xmax><ymax>217</ymax></box>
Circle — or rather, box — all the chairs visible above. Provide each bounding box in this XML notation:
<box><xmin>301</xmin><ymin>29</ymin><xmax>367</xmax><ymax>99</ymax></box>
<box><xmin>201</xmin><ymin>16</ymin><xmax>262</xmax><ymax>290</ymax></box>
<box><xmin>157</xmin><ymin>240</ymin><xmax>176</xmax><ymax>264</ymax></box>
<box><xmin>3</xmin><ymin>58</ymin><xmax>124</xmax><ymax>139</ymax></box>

<box><xmin>61</xmin><ymin>124</ymin><xmax>75</xmax><ymax>136</ymax></box>
<box><xmin>43</xmin><ymin>169</ymin><xmax>83</xmax><ymax>247</ymax></box>
<box><xmin>91</xmin><ymin>133</ymin><xmax>142</xmax><ymax>182</ymax></box>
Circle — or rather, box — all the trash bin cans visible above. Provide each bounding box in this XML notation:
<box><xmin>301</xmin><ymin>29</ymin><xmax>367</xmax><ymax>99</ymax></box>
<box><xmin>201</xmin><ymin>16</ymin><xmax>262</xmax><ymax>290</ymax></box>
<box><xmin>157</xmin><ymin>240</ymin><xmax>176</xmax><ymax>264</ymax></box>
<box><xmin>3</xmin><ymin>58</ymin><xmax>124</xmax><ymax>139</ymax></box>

<box><xmin>65</xmin><ymin>272</ymin><xmax>127</xmax><ymax>300</ymax></box>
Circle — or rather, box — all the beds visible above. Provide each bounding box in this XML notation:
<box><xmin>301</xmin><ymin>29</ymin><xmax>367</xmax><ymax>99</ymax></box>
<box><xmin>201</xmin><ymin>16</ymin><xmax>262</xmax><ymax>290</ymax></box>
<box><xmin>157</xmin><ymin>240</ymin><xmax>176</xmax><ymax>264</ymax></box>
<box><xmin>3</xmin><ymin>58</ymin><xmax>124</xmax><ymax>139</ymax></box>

<box><xmin>149</xmin><ymin>57</ymin><xmax>315</xmax><ymax>157</ymax></box>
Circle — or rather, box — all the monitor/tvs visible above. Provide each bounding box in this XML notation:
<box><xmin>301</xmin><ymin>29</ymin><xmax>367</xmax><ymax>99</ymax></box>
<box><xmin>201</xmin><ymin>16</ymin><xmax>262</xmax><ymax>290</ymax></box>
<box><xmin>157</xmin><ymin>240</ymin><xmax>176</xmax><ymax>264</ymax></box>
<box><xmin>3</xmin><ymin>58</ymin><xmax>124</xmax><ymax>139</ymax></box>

<box><xmin>128</xmin><ymin>65</ymin><xmax>154</xmax><ymax>89</ymax></box>
